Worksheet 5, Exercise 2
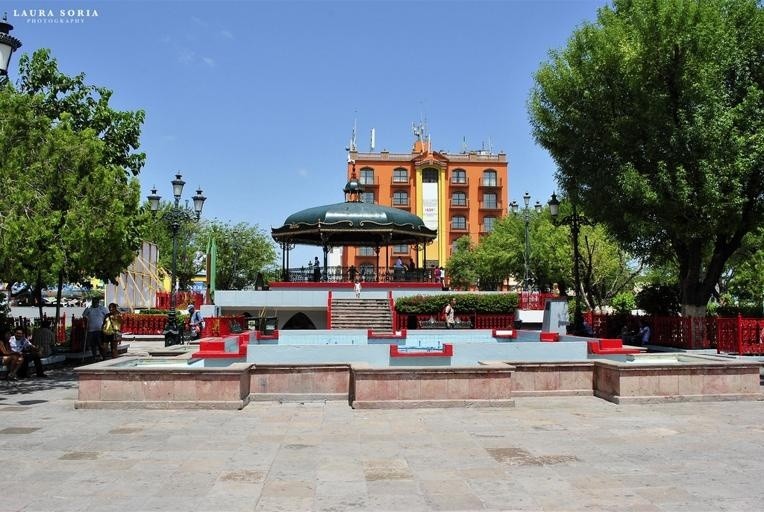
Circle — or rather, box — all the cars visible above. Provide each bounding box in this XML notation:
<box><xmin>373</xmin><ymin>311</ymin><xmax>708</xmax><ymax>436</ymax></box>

<box><xmin>1</xmin><ymin>294</ymin><xmax>80</xmax><ymax>308</ymax></box>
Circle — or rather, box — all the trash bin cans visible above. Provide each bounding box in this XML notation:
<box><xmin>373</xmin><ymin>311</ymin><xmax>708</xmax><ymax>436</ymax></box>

<box><xmin>245</xmin><ymin>317</ymin><xmax>278</xmax><ymax>335</ymax></box>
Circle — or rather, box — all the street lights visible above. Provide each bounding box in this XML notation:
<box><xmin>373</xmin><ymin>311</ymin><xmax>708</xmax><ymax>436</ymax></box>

<box><xmin>299</xmin><ymin>265</ymin><xmax>305</xmax><ymax>272</ymax></box>
<box><xmin>307</xmin><ymin>261</ymin><xmax>312</xmax><ymax>280</ymax></box>
<box><xmin>547</xmin><ymin>184</ymin><xmax>601</xmax><ymax>338</ymax></box>
<box><xmin>508</xmin><ymin>190</ymin><xmax>542</xmax><ymax>291</ymax></box>
<box><xmin>145</xmin><ymin>170</ymin><xmax>209</xmax><ymax>347</ymax></box>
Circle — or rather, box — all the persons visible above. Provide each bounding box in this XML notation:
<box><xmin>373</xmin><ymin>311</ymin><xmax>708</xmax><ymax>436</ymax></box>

<box><xmin>638</xmin><ymin>319</ymin><xmax>651</xmax><ymax>346</ymax></box>
<box><xmin>8</xmin><ymin>326</ymin><xmax>47</xmax><ymax>378</ymax></box>
<box><xmin>352</xmin><ymin>279</ymin><xmax>362</xmax><ymax>298</ymax></box>
<box><xmin>81</xmin><ymin>297</ymin><xmax>109</xmax><ymax>361</ymax></box>
<box><xmin>183</xmin><ymin>304</ymin><xmax>204</xmax><ymax>341</ymax></box>
<box><xmin>100</xmin><ymin>303</ymin><xmax>125</xmax><ymax>359</ymax></box>
<box><xmin>313</xmin><ymin>256</ymin><xmax>321</xmax><ymax>282</ymax></box>
<box><xmin>431</xmin><ymin>264</ymin><xmax>452</xmax><ymax>290</ymax></box>
<box><xmin>546</xmin><ymin>282</ymin><xmax>560</xmax><ymax>296</ymax></box>
<box><xmin>440</xmin><ymin>295</ymin><xmax>456</xmax><ymax>328</ymax></box>
<box><xmin>467</xmin><ymin>283</ymin><xmax>480</xmax><ymax>292</ymax></box>
<box><xmin>0</xmin><ymin>324</ymin><xmax>24</xmax><ymax>380</ymax></box>
<box><xmin>406</xmin><ymin>259</ymin><xmax>415</xmax><ymax>272</ymax></box>
<box><xmin>620</xmin><ymin>320</ymin><xmax>630</xmax><ymax>344</ymax></box>
<box><xmin>396</xmin><ymin>256</ymin><xmax>404</xmax><ymax>273</ymax></box>
<box><xmin>346</xmin><ymin>264</ymin><xmax>358</xmax><ymax>282</ymax></box>
<box><xmin>32</xmin><ymin>319</ymin><xmax>56</xmax><ymax>358</ymax></box>
